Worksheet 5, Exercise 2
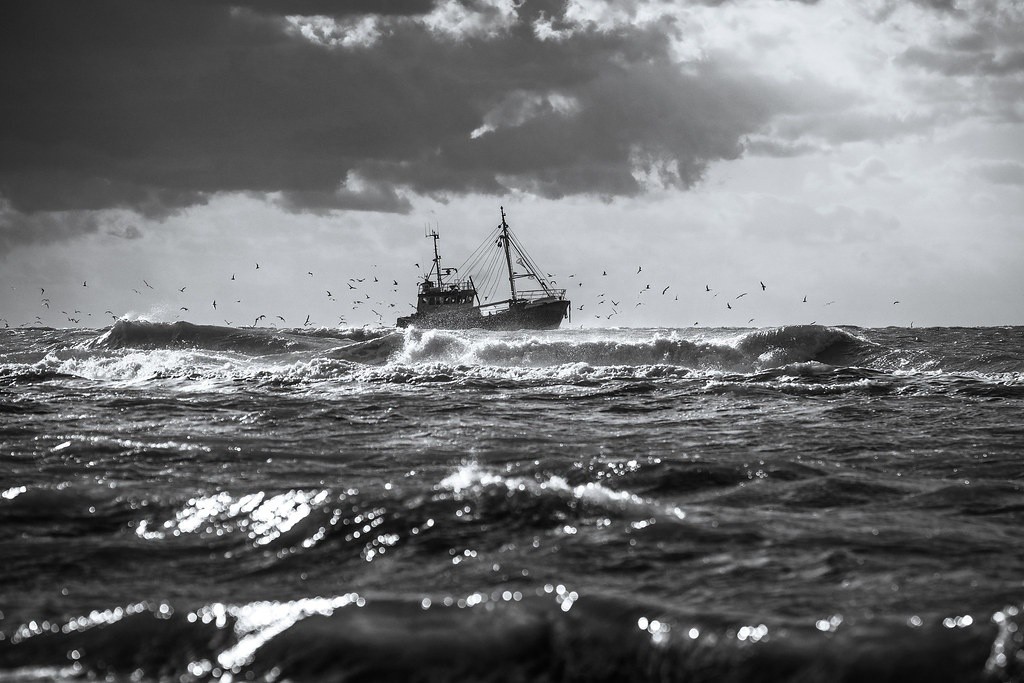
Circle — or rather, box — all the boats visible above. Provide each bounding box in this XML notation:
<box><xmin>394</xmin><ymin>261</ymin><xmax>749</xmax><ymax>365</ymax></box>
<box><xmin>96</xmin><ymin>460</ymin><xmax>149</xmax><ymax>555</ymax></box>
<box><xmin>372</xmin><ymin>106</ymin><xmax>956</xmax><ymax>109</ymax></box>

<box><xmin>394</xmin><ymin>204</ymin><xmax>572</xmax><ymax>332</ymax></box>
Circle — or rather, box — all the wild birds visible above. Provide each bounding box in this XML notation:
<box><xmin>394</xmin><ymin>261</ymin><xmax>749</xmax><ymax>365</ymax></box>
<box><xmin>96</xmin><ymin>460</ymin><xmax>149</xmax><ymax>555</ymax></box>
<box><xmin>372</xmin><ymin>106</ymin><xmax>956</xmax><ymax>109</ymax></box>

<box><xmin>4</xmin><ymin>264</ymin><xmax>921</xmax><ymax>343</ymax></box>
